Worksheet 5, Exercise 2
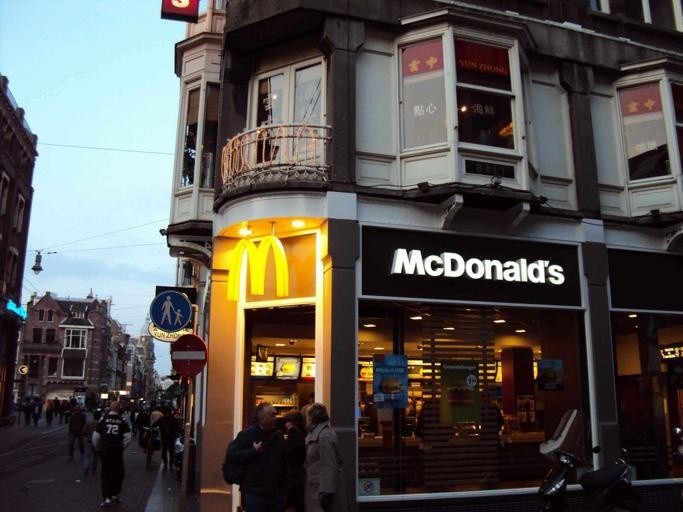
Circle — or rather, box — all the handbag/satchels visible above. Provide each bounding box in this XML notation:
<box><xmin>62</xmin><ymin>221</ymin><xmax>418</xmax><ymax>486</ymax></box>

<box><xmin>223</xmin><ymin>436</ymin><xmax>248</xmax><ymax>485</ymax></box>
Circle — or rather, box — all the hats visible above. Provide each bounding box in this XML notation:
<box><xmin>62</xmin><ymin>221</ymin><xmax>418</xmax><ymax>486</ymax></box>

<box><xmin>276</xmin><ymin>412</ymin><xmax>306</xmax><ymax>434</ymax></box>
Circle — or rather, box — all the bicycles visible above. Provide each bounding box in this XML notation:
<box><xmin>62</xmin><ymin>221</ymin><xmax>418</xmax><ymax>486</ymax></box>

<box><xmin>142</xmin><ymin>425</ymin><xmax>160</xmax><ymax>471</ymax></box>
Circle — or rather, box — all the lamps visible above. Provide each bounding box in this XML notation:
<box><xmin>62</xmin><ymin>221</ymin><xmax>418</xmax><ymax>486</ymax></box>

<box><xmin>25</xmin><ymin>248</ymin><xmax>42</xmax><ymax>275</ymax></box>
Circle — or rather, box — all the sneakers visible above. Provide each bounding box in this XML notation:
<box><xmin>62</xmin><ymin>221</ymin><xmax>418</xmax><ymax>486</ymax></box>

<box><xmin>100</xmin><ymin>495</ymin><xmax>123</xmax><ymax>506</ymax></box>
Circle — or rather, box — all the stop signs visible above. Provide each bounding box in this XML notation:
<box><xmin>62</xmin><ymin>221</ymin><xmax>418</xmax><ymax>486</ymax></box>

<box><xmin>170</xmin><ymin>333</ymin><xmax>208</xmax><ymax>378</ymax></box>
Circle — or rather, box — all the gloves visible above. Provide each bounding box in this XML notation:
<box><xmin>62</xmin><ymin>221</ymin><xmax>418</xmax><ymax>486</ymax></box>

<box><xmin>320</xmin><ymin>492</ymin><xmax>334</xmax><ymax>512</ymax></box>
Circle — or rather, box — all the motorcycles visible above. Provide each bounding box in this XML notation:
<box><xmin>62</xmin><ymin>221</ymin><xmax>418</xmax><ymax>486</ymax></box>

<box><xmin>536</xmin><ymin>409</ymin><xmax>635</xmax><ymax>512</ymax></box>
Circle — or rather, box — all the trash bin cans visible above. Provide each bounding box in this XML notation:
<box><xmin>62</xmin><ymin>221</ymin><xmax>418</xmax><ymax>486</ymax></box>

<box><xmin>179</xmin><ymin>443</ymin><xmax>196</xmax><ymax>473</ymax></box>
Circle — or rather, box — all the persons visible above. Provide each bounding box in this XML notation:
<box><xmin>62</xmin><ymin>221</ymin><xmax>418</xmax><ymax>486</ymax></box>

<box><xmin>160</xmin><ymin>295</ymin><xmax>175</xmax><ymax>326</ymax></box>
<box><xmin>15</xmin><ymin>394</ymin><xmax>178</xmax><ymax>508</ymax></box>
<box><xmin>174</xmin><ymin>309</ymin><xmax>184</xmax><ymax>326</ymax></box>
<box><xmin>414</xmin><ymin>393</ymin><xmax>448</xmax><ymax>445</ymax></box>
<box><xmin>223</xmin><ymin>400</ymin><xmax>343</xmax><ymax>512</ymax></box>
<box><xmin>479</xmin><ymin>389</ymin><xmax>504</xmax><ymax>435</ymax></box>
